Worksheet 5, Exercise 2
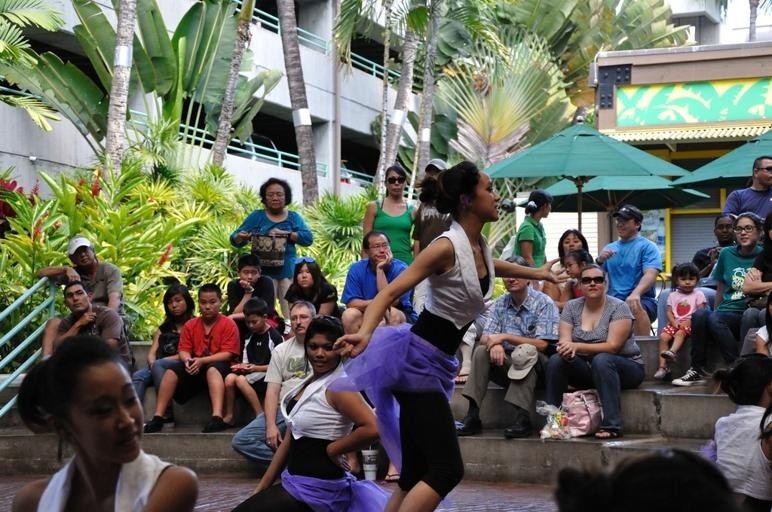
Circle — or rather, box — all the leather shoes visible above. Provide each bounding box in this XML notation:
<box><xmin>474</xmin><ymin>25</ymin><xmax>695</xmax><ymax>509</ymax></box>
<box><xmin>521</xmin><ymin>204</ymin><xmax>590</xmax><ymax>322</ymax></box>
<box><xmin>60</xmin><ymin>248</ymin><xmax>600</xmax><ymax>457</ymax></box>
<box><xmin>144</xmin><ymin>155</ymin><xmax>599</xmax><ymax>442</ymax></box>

<box><xmin>504</xmin><ymin>417</ymin><xmax>533</xmax><ymax>437</ymax></box>
<box><xmin>455</xmin><ymin>414</ymin><xmax>483</xmax><ymax>435</ymax></box>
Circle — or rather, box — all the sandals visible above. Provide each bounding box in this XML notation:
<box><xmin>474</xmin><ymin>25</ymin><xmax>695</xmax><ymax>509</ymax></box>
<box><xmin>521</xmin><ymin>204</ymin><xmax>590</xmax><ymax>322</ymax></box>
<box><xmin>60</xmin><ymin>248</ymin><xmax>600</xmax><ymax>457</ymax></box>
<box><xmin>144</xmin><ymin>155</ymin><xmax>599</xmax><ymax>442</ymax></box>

<box><xmin>596</xmin><ymin>424</ymin><xmax>623</xmax><ymax>439</ymax></box>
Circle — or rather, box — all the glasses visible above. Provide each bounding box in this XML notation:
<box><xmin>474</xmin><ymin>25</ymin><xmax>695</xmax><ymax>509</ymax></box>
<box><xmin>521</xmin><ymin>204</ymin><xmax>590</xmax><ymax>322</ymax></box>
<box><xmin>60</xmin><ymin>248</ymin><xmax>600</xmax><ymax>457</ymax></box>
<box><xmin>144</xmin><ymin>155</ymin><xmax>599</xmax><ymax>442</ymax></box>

<box><xmin>293</xmin><ymin>255</ymin><xmax>315</xmax><ymax>268</ymax></box>
<box><xmin>710</xmin><ymin>223</ymin><xmax>732</xmax><ymax>233</ymax></box>
<box><xmin>756</xmin><ymin>166</ymin><xmax>771</xmax><ymax>171</ymax></box>
<box><xmin>732</xmin><ymin>225</ymin><xmax>758</xmax><ymax>233</ymax></box>
<box><xmin>581</xmin><ymin>276</ymin><xmax>605</xmax><ymax>284</ymax></box>
<box><xmin>386</xmin><ymin>176</ymin><xmax>405</xmax><ymax>184</ymax></box>
<box><xmin>369</xmin><ymin>242</ymin><xmax>390</xmax><ymax>250</ymax></box>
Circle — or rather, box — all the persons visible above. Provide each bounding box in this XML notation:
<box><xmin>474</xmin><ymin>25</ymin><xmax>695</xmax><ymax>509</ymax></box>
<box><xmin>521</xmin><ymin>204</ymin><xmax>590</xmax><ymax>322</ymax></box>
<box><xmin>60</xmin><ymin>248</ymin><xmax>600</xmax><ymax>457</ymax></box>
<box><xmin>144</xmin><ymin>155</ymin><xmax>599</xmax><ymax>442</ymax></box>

<box><xmin>689</xmin><ymin>210</ymin><xmax>739</xmax><ymax>291</ymax></box>
<box><xmin>541</xmin><ymin>266</ymin><xmax>644</xmax><ymax>439</ymax></box>
<box><xmin>509</xmin><ymin>188</ymin><xmax>552</xmax><ymax>271</ymax></box>
<box><xmin>542</xmin><ymin>229</ymin><xmax>588</xmax><ymax>313</ymax></box>
<box><xmin>359</xmin><ymin>163</ymin><xmax>418</xmax><ymax>265</ymax></box>
<box><xmin>284</xmin><ymin>258</ymin><xmax>339</xmax><ymax>323</ymax></box>
<box><xmin>328</xmin><ymin>159</ymin><xmax>573</xmax><ymax>512</ymax></box>
<box><xmin>555</xmin><ymin>448</ymin><xmax>737</xmax><ymax>511</ymax></box>
<box><xmin>410</xmin><ymin>159</ymin><xmax>453</xmax><ymax>262</ymax></box>
<box><xmin>8</xmin><ymin>335</ymin><xmax>199</xmax><ymax>512</ymax></box>
<box><xmin>737</xmin><ymin>214</ymin><xmax>772</xmax><ymax>347</ymax></box>
<box><xmin>35</xmin><ymin>237</ymin><xmax>124</xmax><ymax>360</ymax></box>
<box><xmin>454</xmin><ymin>257</ymin><xmax>561</xmax><ymax>440</ymax></box>
<box><xmin>671</xmin><ymin>211</ymin><xmax>762</xmax><ymax>387</ymax></box>
<box><xmin>232</xmin><ymin>299</ymin><xmax>318</xmax><ymax>466</ymax></box>
<box><xmin>340</xmin><ymin>230</ymin><xmax>413</xmax><ymax>339</ymax></box>
<box><xmin>230</xmin><ymin>315</ymin><xmax>380</xmax><ymax>510</ymax></box>
<box><xmin>231</xmin><ymin>177</ymin><xmax>314</xmax><ymax>335</ymax></box>
<box><xmin>128</xmin><ymin>282</ymin><xmax>196</xmax><ymax>430</ymax></box>
<box><xmin>225</xmin><ymin>253</ymin><xmax>275</xmax><ymax>330</ymax></box>
<box><xmin>51</xmin><ymin>280</ymin><xmax>121</xmax><ymax>358</ymax></box>
<box><xmin>750</xmin><ymin>293</ymin><xmax>772</xmax><ymax>361</ymax></box>
<box><xmin>592</xmin><ymin>203</ymin><xmax>662</xmax><ymax>338</ymax></box>
<box><xmin>563</xmin><ymin>249</ymin><xmax>595</xmax><ymax>300</ymax></box>
<box><xmin>701</xmin><ymin>354</ymin><xmax>772</xmax><ymax>512</ymax></box>
<box><xmin>652</xmin><ymin>263</ymin><xmax>707</xmax><ymax>380</ymax></box>
<box><xmin>721</xmin><ymin>156</ymin><xmax>772</xmax><ymax>230</ymax></box>
<box><xmin>144</xmin><ymin>284</ymin><xmax>240</xmax><ymax>433</ymax></box>
<box><xmin>213</xmin><ymin>298</ymin><xmax>283</xmax><ymax>431</ymax></box>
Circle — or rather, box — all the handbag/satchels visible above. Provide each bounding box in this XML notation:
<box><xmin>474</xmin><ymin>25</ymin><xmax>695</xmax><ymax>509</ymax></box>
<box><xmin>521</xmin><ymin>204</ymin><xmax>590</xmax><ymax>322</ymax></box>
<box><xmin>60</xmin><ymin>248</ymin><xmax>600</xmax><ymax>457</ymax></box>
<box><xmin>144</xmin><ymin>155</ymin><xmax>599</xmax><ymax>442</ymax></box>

<box><xmin>560</xmin><ymin>389</ymin><xmax>605</xmax><ymax>437</ymax></box>
<box><xmin>245</xmin><ymin>220</ymin><xmax>294</xmax><ymax>268</ymax></box>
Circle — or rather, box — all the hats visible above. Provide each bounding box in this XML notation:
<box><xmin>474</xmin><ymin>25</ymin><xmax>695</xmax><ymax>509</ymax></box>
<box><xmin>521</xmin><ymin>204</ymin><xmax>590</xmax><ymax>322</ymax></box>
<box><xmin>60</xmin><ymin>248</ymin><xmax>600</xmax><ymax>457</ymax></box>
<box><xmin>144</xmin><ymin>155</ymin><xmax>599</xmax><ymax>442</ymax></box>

<box><xmin>424</xmin><ymin>158</ymin><xmax>447</xmax><ymax>171</ymax></box>
<box><xmin>612</xmin><ymin>203</ymin><xmax>643</xmax><ymax>223</ymax></box>
<box><xmin>67</xmin><ymin>237</ymin><xmax>91</xmax><ymax>254</ymax></box>
<box><xmin>507</xmin><ymin>342</ymin><xmax>538</xmax><ymax>379</ymax></box>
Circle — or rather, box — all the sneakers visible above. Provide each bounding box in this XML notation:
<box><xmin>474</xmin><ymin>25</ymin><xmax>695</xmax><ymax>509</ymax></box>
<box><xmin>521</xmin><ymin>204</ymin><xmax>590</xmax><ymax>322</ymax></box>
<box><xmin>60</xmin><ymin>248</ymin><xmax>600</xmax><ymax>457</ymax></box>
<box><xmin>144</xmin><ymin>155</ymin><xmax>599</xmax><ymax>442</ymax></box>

<box><xmin>144</xmin><ymin>418</ymin><xmax>175</xmax><ymax>428</ymax></box>
<box><xmin>145</xmin><ymin>417</ymin><xmax>165</xmax><ymax>432</ymax></box>
<box><xmin>202</xmin><ymin>415</ymin><xmax>229</xmax><ymax>433</ymax></box>
<box><xmin>672</xmin><ymin>368</ymin><xmax>707</xmax><ymax>386</ymax></box>
<box><xmin>655</xmin><ymin>366</ymin><xmax>672</xmax><ymax>379</ymax></box>
<box><xmin>662</xmin><ymin>350</ymin><xmax>679</xmax><ymax>364</ymax></box>
